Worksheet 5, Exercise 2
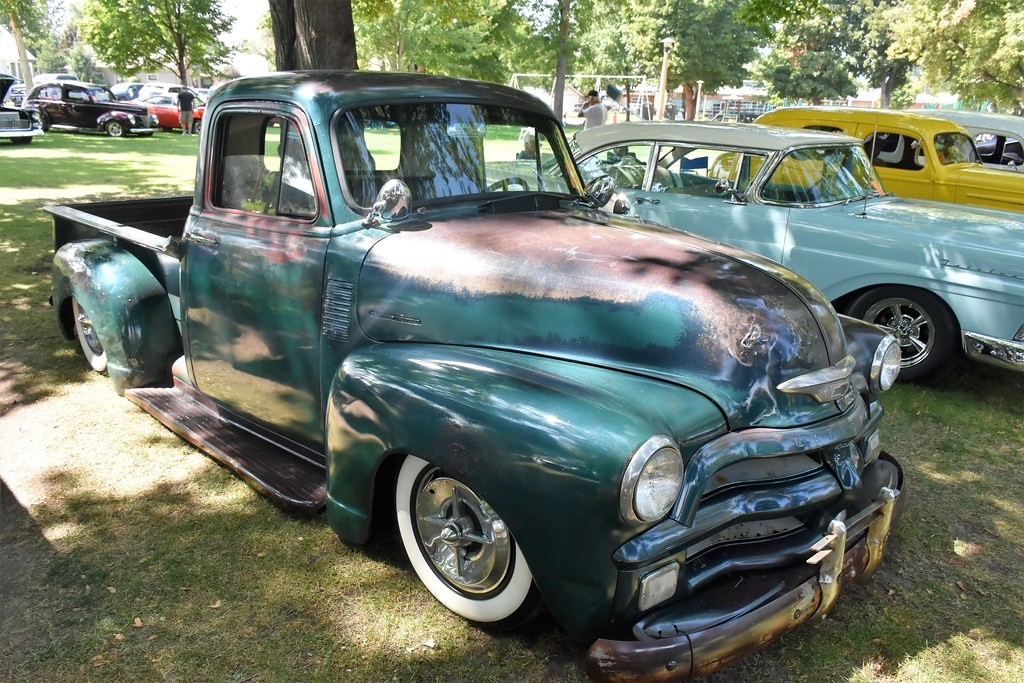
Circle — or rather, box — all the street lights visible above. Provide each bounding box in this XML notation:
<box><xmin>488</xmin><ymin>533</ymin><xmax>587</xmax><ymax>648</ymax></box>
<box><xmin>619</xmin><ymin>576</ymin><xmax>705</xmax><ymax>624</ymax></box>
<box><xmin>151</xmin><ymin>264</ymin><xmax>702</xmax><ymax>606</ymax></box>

<box><xmin>695</xmin><ymin>80</ymin><xmax>704</xmax><ymax>122</ymax></box>
<box><xmin>654</xmin><ymin>37</ymin><xmax>676</xmax><ymax>120</ymax></box>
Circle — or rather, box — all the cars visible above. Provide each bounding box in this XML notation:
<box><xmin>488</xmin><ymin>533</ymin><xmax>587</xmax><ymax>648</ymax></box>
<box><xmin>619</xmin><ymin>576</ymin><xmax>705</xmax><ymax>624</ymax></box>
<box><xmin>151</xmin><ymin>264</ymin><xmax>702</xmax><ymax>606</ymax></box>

<box><xmin>101</xmin><ymin>78</ymin><xmax>281</xmax><ymax>135</ymax></box>
<box><xmin>20</xmin><ymin>78</ymin><xmax>164</xmax><ymax>138</ymax></box>
<box><xmin>0</xmin><ymin>73</ymin><xmax>45</xmax><ymax>144</ymax></box>
<box><xmin>481</xmin><ymin>118</ymin><xmax>1024</xmax><ymax>386</ymax></box>
<box><xmin>841</xmin><ymin>106</ymin><xmax>1024</xmax><ymax>173</ymax></box>
<box><xmin>709</xmin><ymin>104</ymin><xmax>1024</xmax><ymax>215</ymax></box>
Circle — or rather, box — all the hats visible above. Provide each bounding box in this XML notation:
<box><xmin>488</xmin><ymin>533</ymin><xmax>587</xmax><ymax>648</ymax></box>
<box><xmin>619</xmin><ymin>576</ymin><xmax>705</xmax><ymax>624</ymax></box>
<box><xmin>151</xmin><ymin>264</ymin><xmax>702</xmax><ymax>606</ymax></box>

<box><xmin>585</xmin><ymin>90</ymin><xmax>597</xmax><ymax>97</ymax></box>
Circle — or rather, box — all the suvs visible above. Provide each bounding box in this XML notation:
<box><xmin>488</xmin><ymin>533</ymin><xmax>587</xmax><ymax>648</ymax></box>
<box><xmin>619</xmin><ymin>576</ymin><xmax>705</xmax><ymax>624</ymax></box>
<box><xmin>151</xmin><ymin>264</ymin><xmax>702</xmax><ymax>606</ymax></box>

<box><xmin>8</xmin><ymin>72</ymin><xmax>82</xmax><ymax>107</ymax></box>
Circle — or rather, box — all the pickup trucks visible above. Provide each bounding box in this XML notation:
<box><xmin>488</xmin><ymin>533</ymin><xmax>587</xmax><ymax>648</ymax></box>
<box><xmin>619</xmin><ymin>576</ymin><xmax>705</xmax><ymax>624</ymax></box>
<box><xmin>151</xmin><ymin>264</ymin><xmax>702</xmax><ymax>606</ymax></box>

<box><xmin>41</xmin><ymin>69</ymin><xmax>909</xmax><ymax>683</ymax></box>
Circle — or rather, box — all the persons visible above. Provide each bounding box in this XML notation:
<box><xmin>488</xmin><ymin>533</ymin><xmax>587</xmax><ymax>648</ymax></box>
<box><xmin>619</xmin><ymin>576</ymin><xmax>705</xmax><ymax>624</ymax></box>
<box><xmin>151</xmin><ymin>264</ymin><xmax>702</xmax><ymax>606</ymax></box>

<box><xmin>577</xmin><ymin>90</ymin><xmax>608</xmax><ymax>129</ymax></box>
<box><xmin>176</xmin><ymin>85</ymin><xmax>196</xmax><ymax>136</ymax></box>
